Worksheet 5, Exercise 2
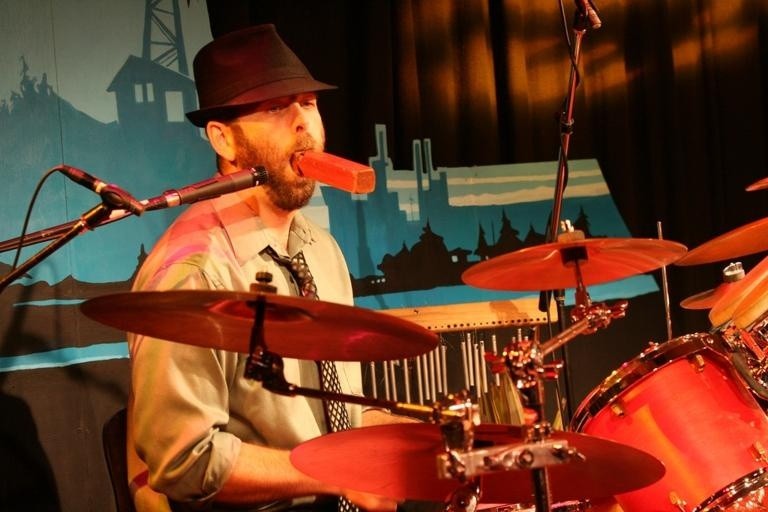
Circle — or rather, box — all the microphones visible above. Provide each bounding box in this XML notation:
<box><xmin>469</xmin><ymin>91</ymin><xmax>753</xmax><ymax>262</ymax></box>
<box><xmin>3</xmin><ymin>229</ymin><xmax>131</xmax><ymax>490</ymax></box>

<box><xmin>61</xmin><ymin>165</ymin><xmax>143</xmax><ymax>218</ymax></box>
<box><xmin>580</xmin><ymin>0</ymin><xmax>602</xmax><ymax>29</ymax></box>
<box><xmin>163</xmin><ymin>164</ymin><xmax>268</xmax><ymax>214</ymax></box>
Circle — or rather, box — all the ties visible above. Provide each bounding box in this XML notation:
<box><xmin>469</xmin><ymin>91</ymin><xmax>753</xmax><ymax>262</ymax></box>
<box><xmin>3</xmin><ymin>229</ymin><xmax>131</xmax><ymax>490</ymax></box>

<box><xmin>266</xmin><ymin>246</ymin><xmax>358</xmax><ymax>510</ymax></box>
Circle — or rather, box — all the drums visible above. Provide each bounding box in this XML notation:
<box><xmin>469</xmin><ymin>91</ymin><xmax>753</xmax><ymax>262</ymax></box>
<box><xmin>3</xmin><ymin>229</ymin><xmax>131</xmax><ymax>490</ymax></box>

<box><xmin>561</xmin><ymin>332</ymin><xmax>768</xmax><ymax>512</ymax></box>
<box><xmin>709</xmin><ymin>257</ymin><xmax>768</xmax><ymax>400</ymax></box>
<box><xmin>733</xmin><ymin>277</ymin><xmax>768</xmax><ymax>389</ymax></box>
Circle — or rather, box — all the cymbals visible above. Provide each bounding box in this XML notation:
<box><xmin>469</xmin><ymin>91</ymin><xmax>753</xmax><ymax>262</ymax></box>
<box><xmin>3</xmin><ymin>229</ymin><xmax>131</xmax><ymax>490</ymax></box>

<box><xmin>76</xmin><ymin>291</ymin><xmax>443</xmax><ymax>361</ymax></box>
<box><xmin>672</xmin><ymin>213</ymin><xmax>767</xmax><ymax>266</ymax></box>
<box><xmin>288</xmin><ymin>424</ymin><xmax>665</xmax><ymax>503</ymax></box>
<box><xmin>679</xmin><ymin>279</ymin><xmax>732</xmax><ymax>316</ymax></box>
<box><xmin>460</xmin><ymin>237</ymin><xmax>687</xmax><ymax>290</ymax></box>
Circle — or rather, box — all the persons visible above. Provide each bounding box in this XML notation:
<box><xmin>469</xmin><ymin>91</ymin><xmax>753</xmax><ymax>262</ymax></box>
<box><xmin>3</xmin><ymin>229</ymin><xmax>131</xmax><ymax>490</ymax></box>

<box><xmin>124</xmin><ymin>18</ymin><xmax>450</xmax><ymax>512</ymax></box>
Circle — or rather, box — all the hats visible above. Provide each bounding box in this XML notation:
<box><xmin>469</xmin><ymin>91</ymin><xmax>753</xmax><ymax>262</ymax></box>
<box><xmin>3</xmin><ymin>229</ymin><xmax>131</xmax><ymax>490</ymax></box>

<box><xmin>185</xmin><ymin>24</ymin><xmax>339</xmax><ymax>128</ymax></box>
